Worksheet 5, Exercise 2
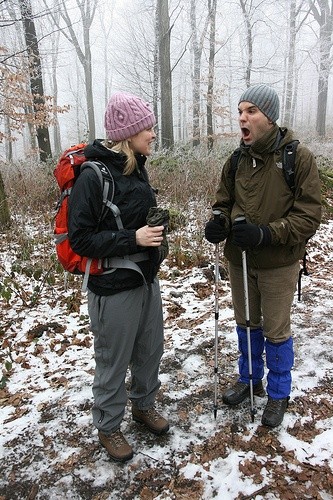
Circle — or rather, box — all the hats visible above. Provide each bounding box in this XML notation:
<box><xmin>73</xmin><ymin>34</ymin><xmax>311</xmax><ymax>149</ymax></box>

<box><xmin>238</xmin><ymin>84</ymin><xmax>279</xmax><ymax>124</ymax></box>
<box><xmin>104</xmin><ymin>94</ymin><xmax>155</xmax><ymax>141</ymax></box>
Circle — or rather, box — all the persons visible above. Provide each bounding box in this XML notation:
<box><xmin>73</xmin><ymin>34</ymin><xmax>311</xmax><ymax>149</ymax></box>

<box><xmin>204</xmin><ymin>85</ymin><xmax>322</xmax><ymax>426</ymax></box>
<box><xmin>66</xmin><ymin>91</ymin><xmax>170</xmax><ymax>462</ymax></box>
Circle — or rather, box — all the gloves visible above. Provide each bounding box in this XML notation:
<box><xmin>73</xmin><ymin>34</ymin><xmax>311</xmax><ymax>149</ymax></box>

<box><xmin>147</xmin><ymin>207</ymin><xmax>169</xmax><ymax>265</ymax></box>
<box><xmin>231</xmin><ymin>224</ymin><xmax>263</xmax><ymax>246</ymax></box>
<box><xmin>205</xmin><ymin>220</ymin><xmax>228</xmax><ymax>244</ymax></box>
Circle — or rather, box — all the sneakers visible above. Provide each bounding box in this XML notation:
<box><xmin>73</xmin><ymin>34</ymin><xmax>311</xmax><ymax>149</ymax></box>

<box><xmin>132</xmin><ymin>405</ymin><xmax>169</xmax><ymax>434</ymax></box>
<box><xmin>221</xmin><ymin>380</ymin><xmax>263</xmax><ymax>405</ymax></box>
<box><xmin>97</xmin><ymin>430</ymin><xmax>133</xmax><ymax>461</ymax></box>
<box><xmin>261</xmin><ymin>394</ymin><xmax>290</xmax><ymax>428</ymax></box>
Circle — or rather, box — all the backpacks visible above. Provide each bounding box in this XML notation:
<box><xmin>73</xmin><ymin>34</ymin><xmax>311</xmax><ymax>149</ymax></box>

<box><xmin>53</xmin><ymin>143</ymin><xmax>102</xmax><ymax>274</ymax></box>
